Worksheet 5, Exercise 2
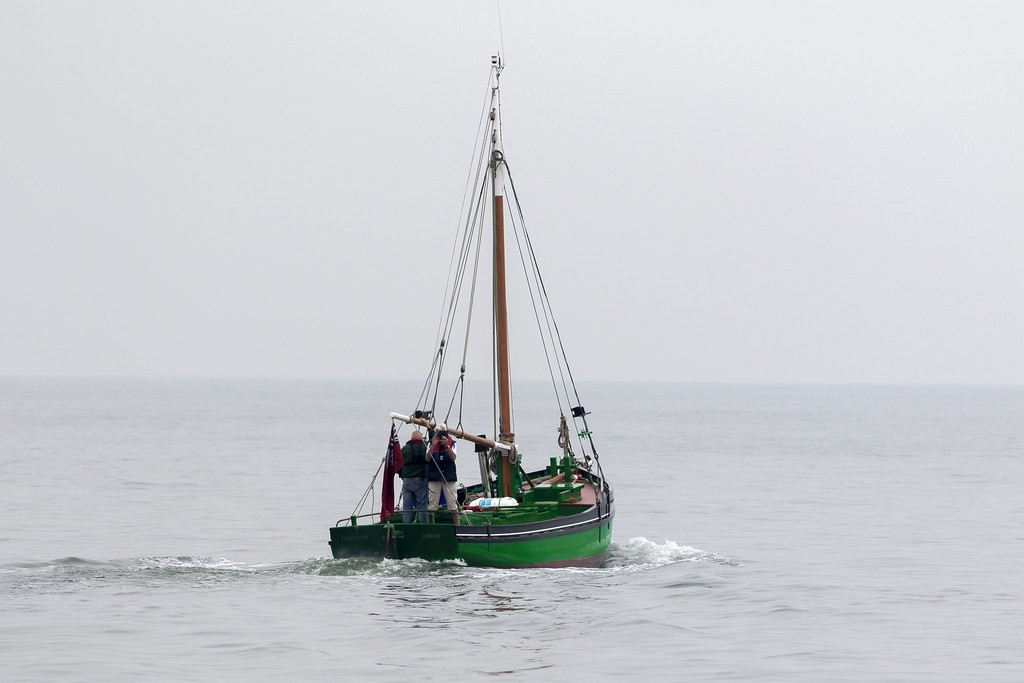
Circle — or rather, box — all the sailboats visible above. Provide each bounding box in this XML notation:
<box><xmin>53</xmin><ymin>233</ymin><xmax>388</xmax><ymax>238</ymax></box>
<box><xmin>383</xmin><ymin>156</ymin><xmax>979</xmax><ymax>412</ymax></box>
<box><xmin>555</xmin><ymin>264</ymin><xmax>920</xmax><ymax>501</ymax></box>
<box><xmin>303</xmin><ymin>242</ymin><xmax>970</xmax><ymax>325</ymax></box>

<box><xmin>328</xmin><ymin>2</ymin><xmax>616</xmax><ymax>568</ymax></box>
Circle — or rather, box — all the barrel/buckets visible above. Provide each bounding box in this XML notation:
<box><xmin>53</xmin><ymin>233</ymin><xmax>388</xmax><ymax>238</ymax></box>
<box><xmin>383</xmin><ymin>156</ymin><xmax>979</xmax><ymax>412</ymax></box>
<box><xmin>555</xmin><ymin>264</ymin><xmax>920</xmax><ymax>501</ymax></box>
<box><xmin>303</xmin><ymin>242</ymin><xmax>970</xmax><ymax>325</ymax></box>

<box><xmin>469</xmin><ymin>496</ymin><xmax>519</xmax><ymax>507</ymax></box>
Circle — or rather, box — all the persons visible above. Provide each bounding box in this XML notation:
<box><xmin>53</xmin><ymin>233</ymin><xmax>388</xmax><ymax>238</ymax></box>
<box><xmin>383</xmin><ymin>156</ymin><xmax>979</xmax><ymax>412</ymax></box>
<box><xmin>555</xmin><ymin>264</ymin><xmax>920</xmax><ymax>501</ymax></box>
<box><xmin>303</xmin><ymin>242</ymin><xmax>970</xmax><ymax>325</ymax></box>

<box><xmin>426</xmin><ymin>423</ymin><xmax>460</xmax><ymax>525</ymax></box>
<box><xmin>397</xmin><ymin>431</ymin><xmax>430</xmax><ymax>523</ymax></box>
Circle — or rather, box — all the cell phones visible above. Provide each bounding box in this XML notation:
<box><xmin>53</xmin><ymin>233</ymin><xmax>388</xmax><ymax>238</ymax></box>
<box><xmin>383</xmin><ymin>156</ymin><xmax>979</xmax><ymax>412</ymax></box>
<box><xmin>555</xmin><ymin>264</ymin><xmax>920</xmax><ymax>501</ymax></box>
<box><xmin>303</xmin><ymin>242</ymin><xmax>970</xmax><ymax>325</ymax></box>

<box><xmin>438</xmin><ymin>431</ymin><xmax>448</xmax><ymax>440</ymax></box>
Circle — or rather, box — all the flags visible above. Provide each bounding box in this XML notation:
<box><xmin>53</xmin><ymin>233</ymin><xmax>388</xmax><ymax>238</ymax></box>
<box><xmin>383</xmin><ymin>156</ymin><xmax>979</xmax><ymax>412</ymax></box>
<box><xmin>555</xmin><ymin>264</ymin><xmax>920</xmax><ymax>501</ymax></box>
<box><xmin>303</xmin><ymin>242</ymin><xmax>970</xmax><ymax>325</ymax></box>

<box><xmin>380</xmin><ymin>423</ymin><xmax>403</xmax><ymax>518</ymax></box>
